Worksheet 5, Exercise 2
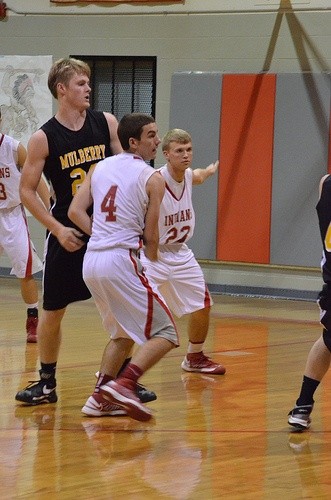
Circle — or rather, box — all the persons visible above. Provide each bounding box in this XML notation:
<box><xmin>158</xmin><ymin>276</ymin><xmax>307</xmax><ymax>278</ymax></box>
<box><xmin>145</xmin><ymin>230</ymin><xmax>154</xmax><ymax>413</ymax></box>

<box><xmin>67</xmin><ymin>114</ymin><xmax>180</xmax><ymax>423</ymax></box>
<box><xmin>15</xmin><ymin>58</ymin><xmax>158</xmax><ymax>406</ymax></box>
<box><xmin>0</xmin><ymin>111</ymin><xmax>50</xmax><ymax>343</ymax></box>
<box><xmin>139</xmin><ymin>128</ymin><xmax>225</xmax><ymax>375</ymax></box>
<box><xmin>285</xmin><ymin>174</ymin><xmax>331</xmax><ymax>430</ymax></box>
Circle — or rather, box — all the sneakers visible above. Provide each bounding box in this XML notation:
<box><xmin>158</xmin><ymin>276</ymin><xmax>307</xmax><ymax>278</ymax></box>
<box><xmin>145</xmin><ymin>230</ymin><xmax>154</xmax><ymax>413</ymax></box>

<box><xmin>287</xmin><ymin>400</ymin><xmax>314</xmax><ymax>430</ymax></box>
<box><xmin>13</xmin><ymin>377</ymin><xmax>58</xmax><ymax>404</ymax></box>
<box><xmin>81</xmin><ymin>378</ymin><xmax>156</xmax><ymax>423</ymax></box>
<box><xmin>26</xmin><ymin>315</ymin><xmax>37</xmax><ymax>342</ymax></box>
<box><xmin>181</xmin><ymin>351</ymin><xmax>227</xmax><ymax>375</ymax></box>
<box><xmin>136</xmin><ymin>385</ymin><xmax>156</xmax><ymax>402</ymax></box>
